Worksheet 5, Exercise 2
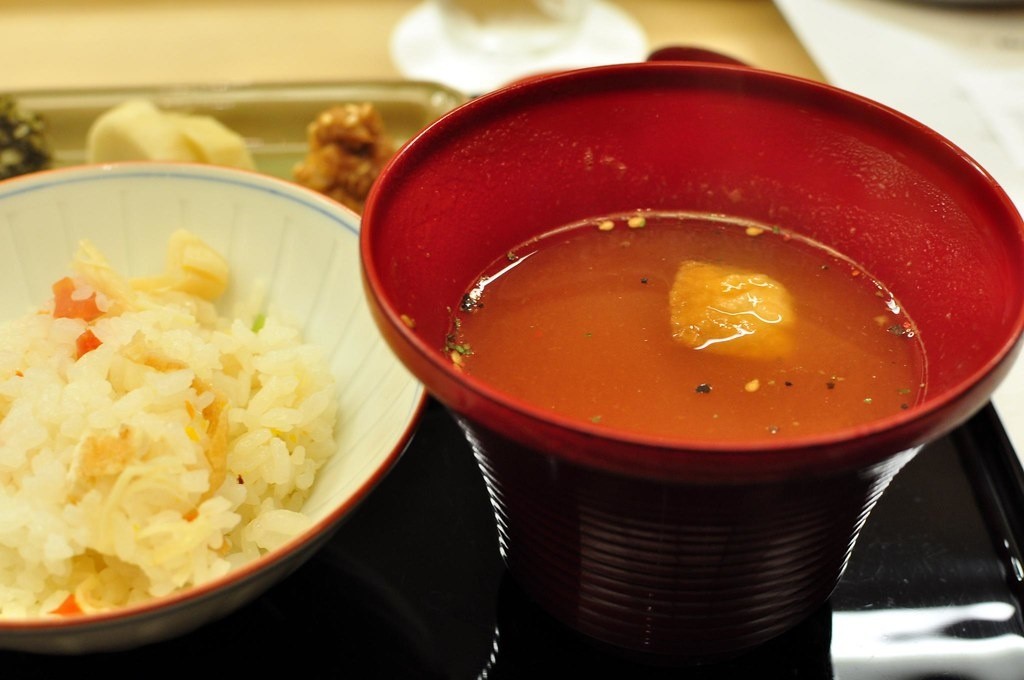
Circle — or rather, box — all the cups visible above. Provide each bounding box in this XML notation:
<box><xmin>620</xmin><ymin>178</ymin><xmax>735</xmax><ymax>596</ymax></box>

<box><xmin>360</xmin><ymin>44</ymin><xmax>1023</xmax><ymax>675</ymax></box>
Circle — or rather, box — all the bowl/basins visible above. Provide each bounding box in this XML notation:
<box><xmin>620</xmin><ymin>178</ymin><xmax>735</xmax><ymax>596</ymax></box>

<box><xmin>0</xmin><ymin>162</ymin><xmax>440</xmax><ymax>653</ymax></box>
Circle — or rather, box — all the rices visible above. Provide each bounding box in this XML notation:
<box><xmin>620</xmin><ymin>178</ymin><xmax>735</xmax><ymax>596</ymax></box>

<box><xmin>1</xmin><ymin>262</ymin><xmax>339</xmax><ymax>617</ymax></box>
